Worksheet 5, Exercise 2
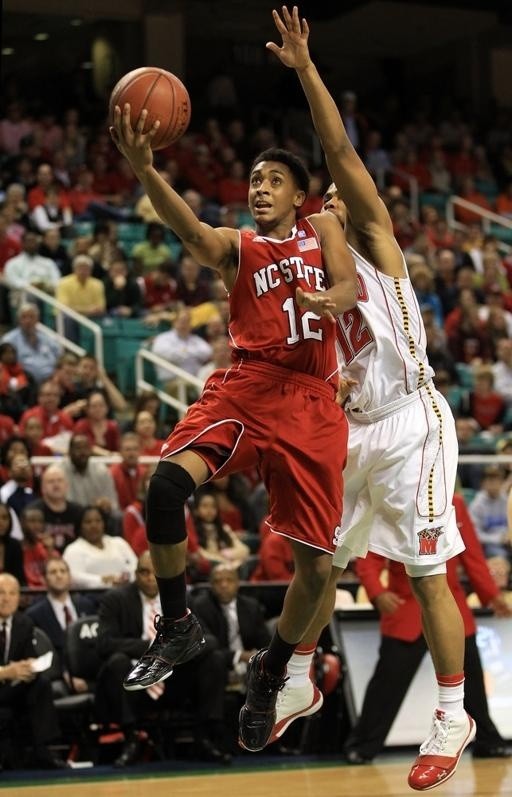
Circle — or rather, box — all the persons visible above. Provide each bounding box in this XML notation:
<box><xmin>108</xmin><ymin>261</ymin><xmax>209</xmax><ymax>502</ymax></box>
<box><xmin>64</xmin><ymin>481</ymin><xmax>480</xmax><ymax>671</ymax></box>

<box><xmin>1</xmin><ymin>228</ymin><xmax>292</xmax><ymax>769</ymax></box>
<box><xmin>266</xmin><ymin>5</ymin><xmax>479</xmax><ymax>790</ymax></box>
<box><xmin>109</xmin><ymin>104</ymin><xmax>359</xmax><ymax>752</ymax></box>
<box><xmin>1</xmin><ymin>92</ymin><xmax>512</xmax><ymax>232</ymax></box>
<box><xmin>393</xmin><ymin>202</ymin><xmax>511</xmax><ymax>615</ymax></box>
<box><xmin>348</xmin><ymin>501</ymin><xmax>511</xmax><ymax>770</ymax></box>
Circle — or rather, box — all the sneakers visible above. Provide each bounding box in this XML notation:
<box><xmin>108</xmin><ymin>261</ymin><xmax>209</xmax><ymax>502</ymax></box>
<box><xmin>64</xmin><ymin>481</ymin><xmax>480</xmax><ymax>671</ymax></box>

<box><xmin>238</xmin><ymin>678</ymin><xmax>323</xmax><ymax>751</ymax></box>
<box><xmin>122</xmin><ymin>606</ymin><xmax>208</xmax><ymax>692</ymax></box>
<box><xmin>238</xmin><ymin>647</ymin><xmax>290</xmax><ymax>753</ymax></box>
<box><xmin>407</xmin><ymin>707</ymin><xmax>477</xmax><ymax>790</ymax></box>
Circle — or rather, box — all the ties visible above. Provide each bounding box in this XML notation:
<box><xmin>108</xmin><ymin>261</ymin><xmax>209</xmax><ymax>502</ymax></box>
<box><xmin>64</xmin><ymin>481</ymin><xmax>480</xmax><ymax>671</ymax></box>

<box><xmin>147</xmin><ymin>597</ymin><xmax>158</xmax><ymax>644</ymax></box>
<box><xmin>0</xmin><ymin>620</ymin><xmax>6</xmax><ymax>666</ymax></box>
<box><xmin>63</xmin><ymin>604</ymin><xmax>73</xmax><ymax>629</ymax></box>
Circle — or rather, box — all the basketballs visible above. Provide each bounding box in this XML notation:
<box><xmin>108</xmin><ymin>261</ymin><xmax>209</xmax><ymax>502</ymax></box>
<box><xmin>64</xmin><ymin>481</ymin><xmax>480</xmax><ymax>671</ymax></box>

<box><xmin>108</xmin><ymin>64</ymin><xmax>193</xmax><ymax>152</ymax></box>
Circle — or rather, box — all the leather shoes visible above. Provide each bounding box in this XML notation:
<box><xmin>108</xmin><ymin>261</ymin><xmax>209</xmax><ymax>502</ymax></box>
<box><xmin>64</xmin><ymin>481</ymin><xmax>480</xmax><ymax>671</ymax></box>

<box><xmin>195</xmin><ymin>737</ymin><xmax>232</xmax><ymax>765</ymax></box>
<box><xmin>114</xmin><ymin>742</ymin><xmax>144</xmax><ymax>768</ymax></box>
<box><xmin>347</xmin><ymin>750</ymin><xmax>364</xmax><ymax>765</ymax></box>
<box><xmin>473</xmin><ymin>742</ymin><xmax>512</xmax><ymax>757</ymax></box>
